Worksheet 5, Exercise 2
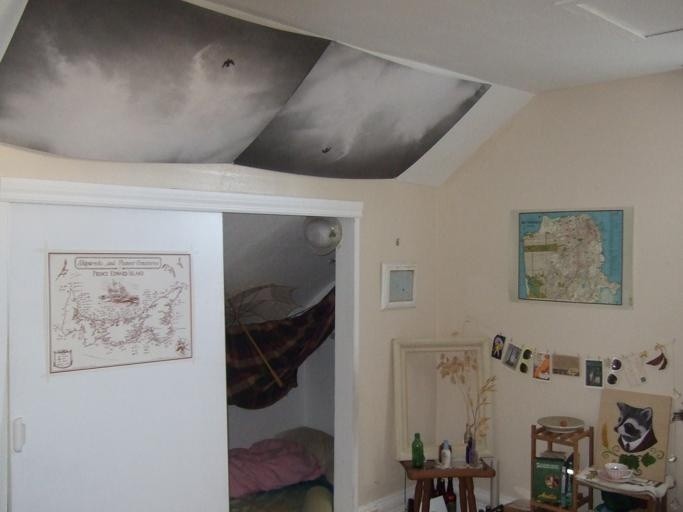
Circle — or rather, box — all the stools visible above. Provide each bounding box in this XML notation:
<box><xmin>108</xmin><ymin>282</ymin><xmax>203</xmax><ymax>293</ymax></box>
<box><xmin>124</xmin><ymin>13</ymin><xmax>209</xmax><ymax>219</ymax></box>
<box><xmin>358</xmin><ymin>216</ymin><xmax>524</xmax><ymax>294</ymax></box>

<box><xmin>399</xmin><ymin>458</ymin><xmax>498</xmax><ymax>512</ymax></box>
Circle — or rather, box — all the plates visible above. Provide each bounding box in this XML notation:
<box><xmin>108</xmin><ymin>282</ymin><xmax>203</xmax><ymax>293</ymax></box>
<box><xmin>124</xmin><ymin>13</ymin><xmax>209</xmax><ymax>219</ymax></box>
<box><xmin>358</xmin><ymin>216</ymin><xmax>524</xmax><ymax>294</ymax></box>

<box><xmin>598</xmin><ymin>469</ymin><xmax>633</xmax><ymax>484</ymax></box>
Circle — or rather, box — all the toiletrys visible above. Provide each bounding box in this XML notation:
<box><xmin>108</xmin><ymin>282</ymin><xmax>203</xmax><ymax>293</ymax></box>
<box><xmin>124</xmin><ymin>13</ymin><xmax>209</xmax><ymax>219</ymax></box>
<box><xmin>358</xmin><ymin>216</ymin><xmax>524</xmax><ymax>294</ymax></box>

<box><xmin>439</xmin><ymin>439</ymin><xmax>451</xmax><ymax>463</ymax></box>
<box><xmin>441</xmin><ymin>440</ymin><xmax>452</xmax><ymax>467</ymax></box>
<box><xmin>466</xmin><ymin>437</ymin><xmax>473</xmax><ymax>464</ymax></box>
<box><xmin>468</xmin><ymin>440</ymin><xmax>477</xmax><ymax>465</ymax></box>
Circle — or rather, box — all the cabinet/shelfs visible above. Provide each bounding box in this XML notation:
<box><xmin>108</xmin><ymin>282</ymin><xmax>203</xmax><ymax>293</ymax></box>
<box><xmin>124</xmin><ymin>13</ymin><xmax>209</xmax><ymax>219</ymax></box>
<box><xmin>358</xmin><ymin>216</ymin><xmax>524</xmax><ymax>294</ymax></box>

<box><xmin>529</xmin><ymin>425</ymin><xmax>666</xmax><ymax>512</ymax></box>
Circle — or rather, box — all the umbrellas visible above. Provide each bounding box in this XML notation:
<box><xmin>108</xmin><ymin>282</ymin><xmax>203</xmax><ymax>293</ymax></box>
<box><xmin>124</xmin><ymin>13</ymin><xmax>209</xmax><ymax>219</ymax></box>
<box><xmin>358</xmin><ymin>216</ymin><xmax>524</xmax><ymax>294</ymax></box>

<box><xmin>225</xmin><ymin>278</ymin><xmax>302</xmax><ymax>388</ymax></box>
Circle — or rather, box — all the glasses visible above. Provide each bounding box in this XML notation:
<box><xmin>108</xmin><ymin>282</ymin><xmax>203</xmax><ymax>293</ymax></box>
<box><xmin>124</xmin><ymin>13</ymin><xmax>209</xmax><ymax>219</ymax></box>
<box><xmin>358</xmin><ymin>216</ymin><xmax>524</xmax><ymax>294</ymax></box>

<box><xmin>519</xmin><ymin>348</ymin><xmax>532</xmax><ymax>373</ymax></box>
<box><xmin>606</xmin><ymin>356</ymin><xmax>621</xmax><ymax>385</ymax></box>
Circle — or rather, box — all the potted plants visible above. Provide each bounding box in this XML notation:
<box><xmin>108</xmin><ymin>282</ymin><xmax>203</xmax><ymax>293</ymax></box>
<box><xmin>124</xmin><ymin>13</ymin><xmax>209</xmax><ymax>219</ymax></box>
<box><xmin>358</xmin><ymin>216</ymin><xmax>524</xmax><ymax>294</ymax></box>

<box><xmin>436</xmin><ymin>353</ymin><xmax>498</xmax><ymax>468</ymax></box>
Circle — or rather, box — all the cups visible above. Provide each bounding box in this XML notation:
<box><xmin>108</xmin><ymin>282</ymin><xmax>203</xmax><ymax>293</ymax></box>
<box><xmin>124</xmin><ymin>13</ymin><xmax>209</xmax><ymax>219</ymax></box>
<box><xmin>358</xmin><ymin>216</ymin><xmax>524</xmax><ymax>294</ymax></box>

<box><xmin>604</xmin><ymin>463</ymin><xmax>633</xmax><ymax>480</ymax></box>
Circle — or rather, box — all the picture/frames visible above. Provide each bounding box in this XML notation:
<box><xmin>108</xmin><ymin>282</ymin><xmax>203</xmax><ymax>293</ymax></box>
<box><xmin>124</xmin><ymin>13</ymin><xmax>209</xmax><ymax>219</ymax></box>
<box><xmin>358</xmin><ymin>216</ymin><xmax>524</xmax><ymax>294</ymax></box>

<box><xmin>379</xmin><ymin>263</ymin><xmax>417</xmax><ymax>309</ymax></box>
<box><xmin>509</xmin><ymin>205</ymin><xmax>634</xmax><ymax>311</ymax></box>
<box><xmin>391</xmin><ymin>338</ymin><xmax>495</xmax><ymax>462</ymax></box>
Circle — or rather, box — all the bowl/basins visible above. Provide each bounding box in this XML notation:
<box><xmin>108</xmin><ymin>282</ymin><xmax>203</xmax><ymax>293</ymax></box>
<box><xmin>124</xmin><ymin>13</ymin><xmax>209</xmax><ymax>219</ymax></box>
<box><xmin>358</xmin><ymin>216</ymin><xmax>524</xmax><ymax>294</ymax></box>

<box><xmin>537</xmin><ymin>416</ymin><xmax>586</xmax><ymax>434</ymax></box>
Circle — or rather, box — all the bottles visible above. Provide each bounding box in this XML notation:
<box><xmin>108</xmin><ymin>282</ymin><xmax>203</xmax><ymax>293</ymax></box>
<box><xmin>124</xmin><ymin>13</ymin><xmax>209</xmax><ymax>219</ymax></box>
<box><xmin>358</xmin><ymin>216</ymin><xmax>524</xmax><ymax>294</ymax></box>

<box><xmin>411</xmin><ymin>432</ymin><xmax>424</xmax><ymax>468</ymax></box>
<box><xmin>434</xmin><ymin>477</ymin><xmax>441</xmax><ymax>496</ymax></box>
<box><xmin>443</xmin><ymin>476</ymin><xmax>456</xmax><ymax>512</ymax></box>
<box><xmin>440</xmin><ymin>479</ymin><xmax>446</xmax><ymax>495</ymax></box>
